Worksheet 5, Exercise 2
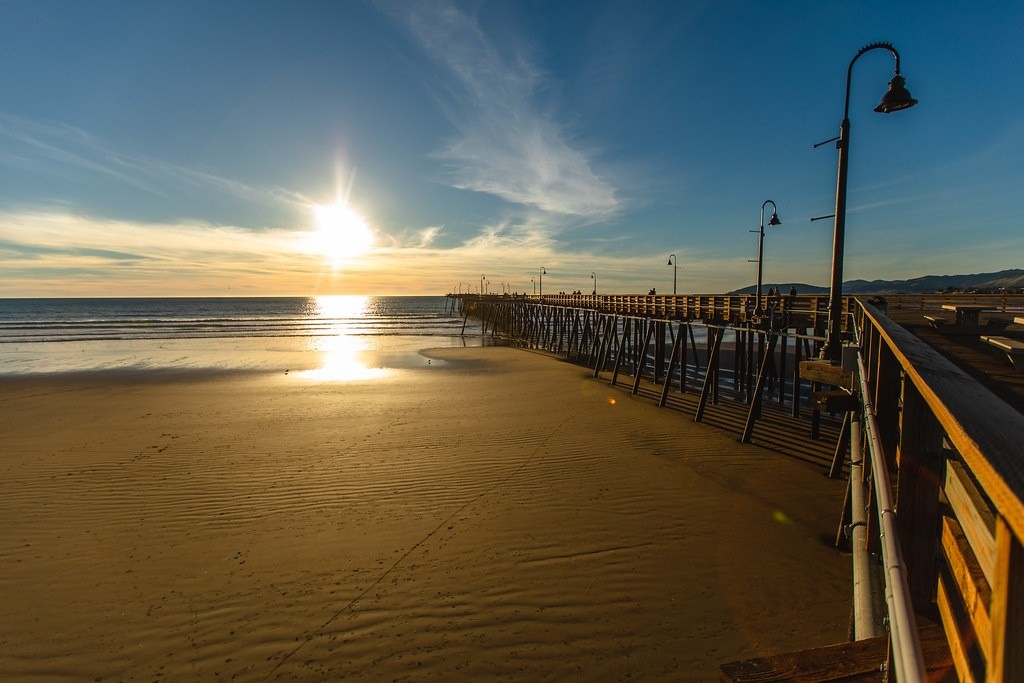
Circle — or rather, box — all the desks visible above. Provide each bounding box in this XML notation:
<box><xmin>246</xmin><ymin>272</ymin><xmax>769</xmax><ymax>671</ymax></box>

<box><xmin>942</xmin><ymin>303</ymin><xmax>997</xmax><ymax>329</ymax></box>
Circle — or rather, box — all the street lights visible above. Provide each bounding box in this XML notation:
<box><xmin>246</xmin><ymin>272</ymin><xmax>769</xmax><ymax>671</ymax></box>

<box><xmin>539</xmin><ymin>266</ymin><xmax>546</xmax><ymax>304</ymax></box>
<box><xmin>480</xmin><ymin>274</ymin><xmax>485</xmax><ymax>295</ymax></box>
<box><xmin>507</xmin><ymin>283</ymin><xmax>510</xmax><ymax>294</ymax></box>
<box><xmin>531</xmin><ymin>276</ymin><xmax>536</xmax><ymax>295</ymax></box>
<box><xmin>816</xmin><ymin>40</ymin><xmax>918</xmax><ymax>360</ymax></box>
<box><xmin>501</xmin><ymin>281</ymin><xmax>504</xmax><ymax>294</ymax></box>
<box><xmin>667</xmin><ymin>253</ymin><xmax>677</xmax><ymax>294</ymax></box>
<box><xmin>591</xmin><ymin>272</ymin><xmax>597</xmax><ymax>294</ymax></box>
<box><xmin>753</xmin><ymin>199</ymin><xmax>782</xmax><ymax>316</ymax></box>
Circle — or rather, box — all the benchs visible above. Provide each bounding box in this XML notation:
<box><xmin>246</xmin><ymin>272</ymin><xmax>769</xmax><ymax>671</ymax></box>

<box><xmin>1014</xmin><ymin>316</ymin><xmax>1024</xmax><ymax>325</ymax></box>
<box><xmin>985</xmin><ymin>315</ymin><xmax>1013</xmax><ymax>333</ymax></box>
<box><xmin>980</xmin><ymin>335</ymin><xmax>1024</xmax><ymax>369</ymax></box>
<box><xmin>924</xmin><ymin>315</ymin><xmax>948</xmax><ymax>330</ymax></box>
